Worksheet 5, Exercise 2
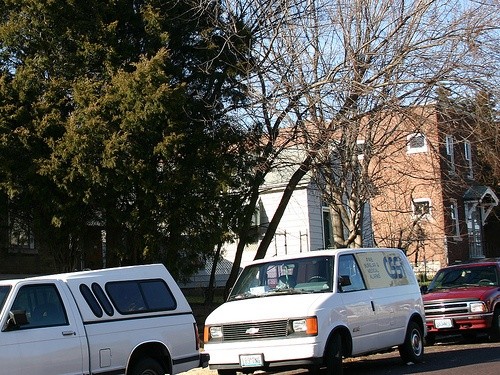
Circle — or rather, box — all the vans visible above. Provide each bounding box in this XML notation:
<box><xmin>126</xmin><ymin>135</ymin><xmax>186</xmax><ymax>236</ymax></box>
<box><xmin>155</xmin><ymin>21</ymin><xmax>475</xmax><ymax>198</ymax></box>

<box><xmin>0</xmin><ymin>264</ymin><xmax>210</xmax><ymax>375</ymax></box>
<box><xmin>204</xmin><ymin>247</ymin><xmax>427</xmax><ymax>375</ymax></box>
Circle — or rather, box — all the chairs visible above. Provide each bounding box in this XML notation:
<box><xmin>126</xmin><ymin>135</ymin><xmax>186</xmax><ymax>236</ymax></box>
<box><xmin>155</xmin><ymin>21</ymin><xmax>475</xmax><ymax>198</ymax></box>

<box><xmin>278</xmin><ymin>275</ymin><xmax>296</xmax><ymax>288</ymax></box>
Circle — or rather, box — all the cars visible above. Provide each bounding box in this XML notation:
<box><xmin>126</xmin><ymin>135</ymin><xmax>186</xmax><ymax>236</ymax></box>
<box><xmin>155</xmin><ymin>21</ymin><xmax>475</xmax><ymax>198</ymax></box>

<box><xmin>421</xmin><ymin>261</ymin><xmax>500</xmax><ymax>346</ymax></box>
